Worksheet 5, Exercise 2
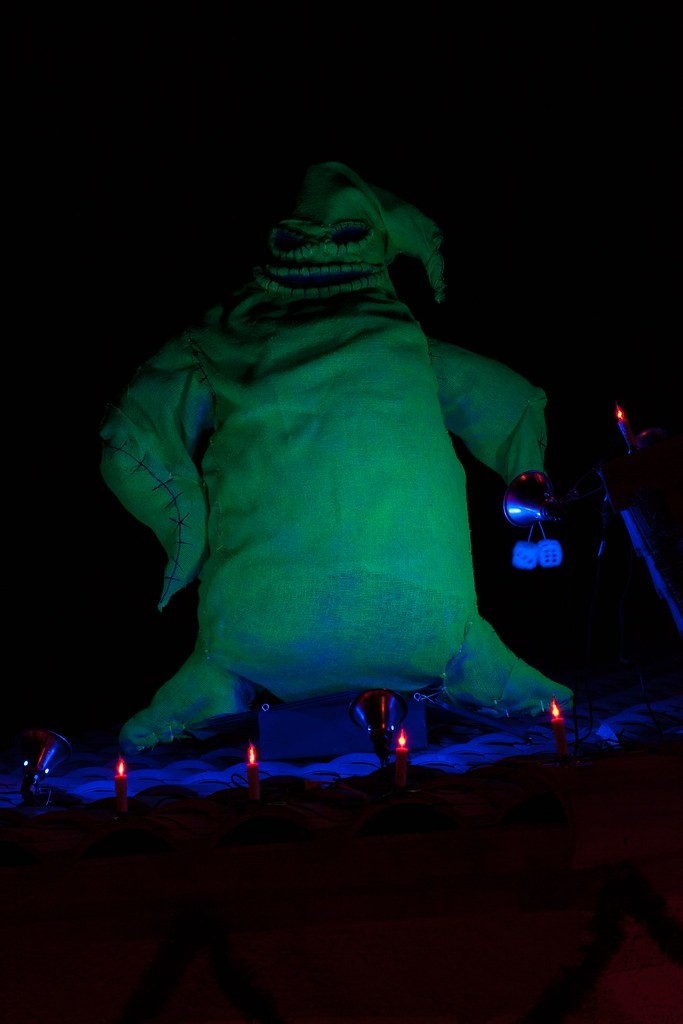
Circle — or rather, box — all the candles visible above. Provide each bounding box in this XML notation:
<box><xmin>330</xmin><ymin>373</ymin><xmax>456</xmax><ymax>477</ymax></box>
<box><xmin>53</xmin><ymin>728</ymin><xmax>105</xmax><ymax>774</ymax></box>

<box><xmin>112</xmin><ymin>755</ymin><xmax>129</xmax><ymax>815</ymax></box>
<box><xmin>615</xmin><ymin>403</ymin><xmax>634</xmax><ymax>447</ymax></box>
<box><xmin>394</xmin><ymin>725</ymin><xmax>411</xmax><ymax>787</ymax></box>
<box><xmin>245</xmin><ymin>740</ymin><xmax>261</xmax><ymax>802</ymax></box>
<box><xmin>550</xmin><ymin>698</ymin><xmax>567</xmax><ymax>758</ymax></box>
